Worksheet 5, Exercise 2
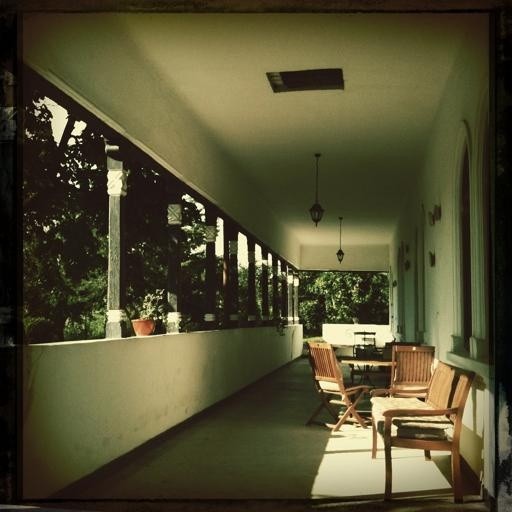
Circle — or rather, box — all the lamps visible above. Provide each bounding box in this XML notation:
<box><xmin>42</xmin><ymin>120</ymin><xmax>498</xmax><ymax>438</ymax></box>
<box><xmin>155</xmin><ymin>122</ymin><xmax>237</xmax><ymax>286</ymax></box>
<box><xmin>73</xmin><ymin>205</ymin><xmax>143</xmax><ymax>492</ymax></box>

<box><xmin>309</xmin><ymin>153</ymin><xmax>325</xmax><ymax>227</ymax></box>
<box><xmin>336</xmin><ymin>217</ymin><xmax>344</xmax><ymax>264</ymax></box>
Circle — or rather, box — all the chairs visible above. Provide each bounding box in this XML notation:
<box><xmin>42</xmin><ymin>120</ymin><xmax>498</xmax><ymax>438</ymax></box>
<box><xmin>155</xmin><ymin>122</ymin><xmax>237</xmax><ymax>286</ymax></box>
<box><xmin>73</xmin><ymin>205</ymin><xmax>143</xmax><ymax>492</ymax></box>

<box><xmin>305</xmin><ymin>331</ymin><xmax>475</xmax><ymax>502</ymax></box>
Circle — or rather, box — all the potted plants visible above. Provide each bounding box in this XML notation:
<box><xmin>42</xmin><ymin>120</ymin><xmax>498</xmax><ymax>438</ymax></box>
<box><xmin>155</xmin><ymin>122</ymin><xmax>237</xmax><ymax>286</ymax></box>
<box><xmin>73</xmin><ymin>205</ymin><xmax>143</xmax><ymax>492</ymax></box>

<box><xmin>130</xmin><ymin>288</ymin><xmax>166</xmax><ymax>336</ymax></box>
